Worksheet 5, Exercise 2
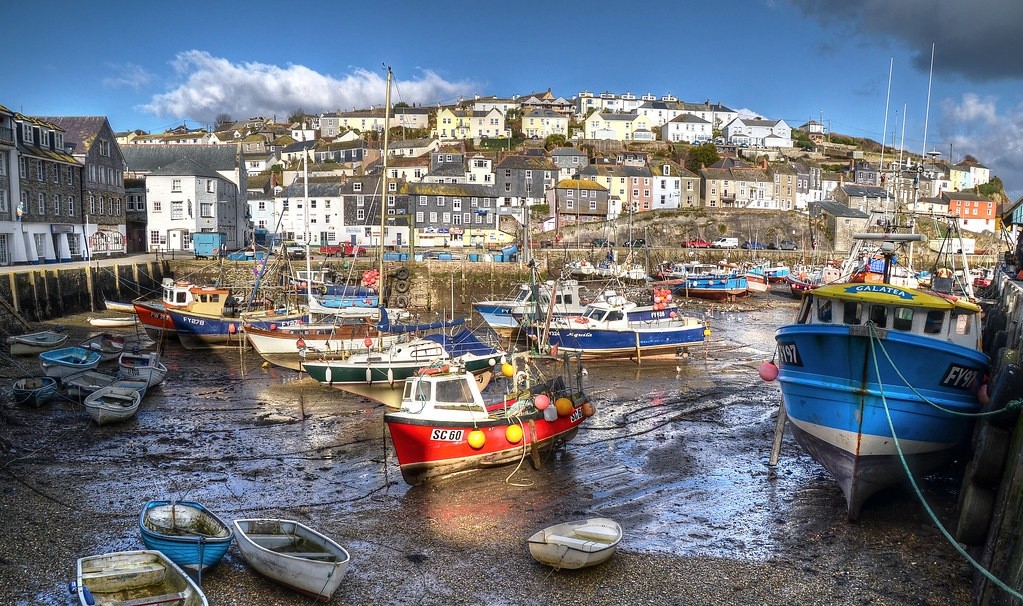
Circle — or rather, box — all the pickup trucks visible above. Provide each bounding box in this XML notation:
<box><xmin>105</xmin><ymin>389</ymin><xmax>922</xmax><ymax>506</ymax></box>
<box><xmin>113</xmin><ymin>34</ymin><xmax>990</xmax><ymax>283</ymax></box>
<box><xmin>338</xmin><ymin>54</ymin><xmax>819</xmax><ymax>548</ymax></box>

<box><xmin>320</xmin><ymin>241</ymin><xmax>366</xmax><ymax>258</ymax></box>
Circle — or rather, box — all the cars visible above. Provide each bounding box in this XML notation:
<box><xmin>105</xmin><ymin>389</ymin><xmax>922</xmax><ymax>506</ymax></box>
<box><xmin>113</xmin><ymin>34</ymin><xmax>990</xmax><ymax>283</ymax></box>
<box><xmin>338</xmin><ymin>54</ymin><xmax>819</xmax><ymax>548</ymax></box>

<box><xmin>622</xmin><ymin>238</ymin><xmax>645</xmax><ymax>248</ymax></box>
<box><xmin>593</xmin><ymin>238</ymin><xmax>615</xmax><ymax>248</ymax></box>
<box><xmin>274</xmin><ymin>240</ymin><xmax>306</xmax><ymax>261</ymax></box>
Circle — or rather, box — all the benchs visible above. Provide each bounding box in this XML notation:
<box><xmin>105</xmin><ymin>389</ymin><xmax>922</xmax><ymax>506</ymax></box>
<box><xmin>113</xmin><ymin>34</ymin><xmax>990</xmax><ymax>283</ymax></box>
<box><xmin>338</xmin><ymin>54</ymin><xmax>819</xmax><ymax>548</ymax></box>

<box><xmin>96</xmin><ymin>591</ymin><xmax>182</xmax><ymax>606</ymax></box>
<box><xmin>281</xmin><ymin>551</ymin><xmax>332</xmax><ymax>558</ymax></box>
<box><xmin>102</xmin><ymin>393</ymin><xmax>134</xmax><ymax>400</ymax></box>
<box><xmin>82</xmin><ymin>562</ymin><xmax>166</xmax><ymax>591</ymax></box>
<box><xmin>246</xmin><ymin>532</ymin><xmax>300</xmax><ymax>545</ymax></box>
<box><xmin>548</xmin><ymin>535</ymin><xmax>607</xmax><ymax>548</ymax></box>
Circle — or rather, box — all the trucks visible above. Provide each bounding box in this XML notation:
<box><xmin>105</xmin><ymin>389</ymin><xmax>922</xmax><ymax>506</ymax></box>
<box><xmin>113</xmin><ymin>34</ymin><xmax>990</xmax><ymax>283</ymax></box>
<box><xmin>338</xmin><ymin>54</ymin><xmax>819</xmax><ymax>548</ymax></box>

<box><xmin>191</xmin><ymin>231</ymin><xmax>227</xmax><ymax>260</ymax></box>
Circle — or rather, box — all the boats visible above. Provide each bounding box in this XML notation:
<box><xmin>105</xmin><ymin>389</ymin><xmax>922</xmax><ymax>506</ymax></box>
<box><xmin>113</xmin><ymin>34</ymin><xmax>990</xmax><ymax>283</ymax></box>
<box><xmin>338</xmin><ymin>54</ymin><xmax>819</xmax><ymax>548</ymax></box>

<box><xmin>233</xmin><ymin>519</ymin><xmax>351</xmax><ymax>603</ymax></box>
<box><xmin>118</xmin><ymin>345</ymin><xmax>169</xmax><ymax>389</ymax></box>
<box><xmin>84</xmin><ymin>386</ymin><xmax>140</xmax><ymax>427</ymax></box>
<box><xmin>139</xmin><ymin>499</ymin><xmax>234</xmax><ymax>587</ymax></box>
<box><xmin>86</xmin><ymin>316</ymin><xmax>140</xmax><ymax>327</ymax></box>
<box><xmin>61</xmin><ymin>368</ymin><xmax>118</xmax><ymax>397</ymax></box>
<box><xmin>37</xmin><ymin>342</ymin><xmax>102</xmax><ymax>379</ymax></box>
<box><xmin>76</xmin><ymin>550</ymin><xmax>209</xmax><ymax>606</ymax></box>
<box><xmin>769</xmin><ymin>43</ymin><xmax>995</xmax><ymax>525</ymax></box>
<box><xmin>526</xmin><ymin>516</ymin><xmax>623</xmax><ymax>572</ymax></box>
<box><xmin>382</xmin><ymin>308</ymin><xmax>593</xmax><ymax>487</ymax></box>
<box><xmin>107</xmin><ymin>378</ymin><xmax>148</xmax><ymax>401</ymax></box>
<box><xmin>103</xmin><ymin>298</ymin><xmax>137</xmax><ymax>314</ymax></box>
<box><xmin>6</xmin><ymin>324</ymin><xmax>70</xmax><ymax>356</ymax></box>
<box><xmin>78</xmin><ymin>332</ymin><xmax>127</xmax><ymax>362</ymax></box>
<box><xmin>12</xmin><ymin>377</ymin><xmax>58</xmax><ymax>409</ymax></box>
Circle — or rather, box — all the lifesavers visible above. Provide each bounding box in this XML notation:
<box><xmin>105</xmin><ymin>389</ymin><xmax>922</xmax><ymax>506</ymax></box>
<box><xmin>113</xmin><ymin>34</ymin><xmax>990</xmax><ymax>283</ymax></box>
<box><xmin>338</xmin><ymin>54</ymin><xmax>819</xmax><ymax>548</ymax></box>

<box><xmin>396</xmin><ymin>295</ymin><xmax>407</xmax><ymax>308</ymax></box>
<box><xmin>176</xmin><ymin>282</ymin><xmax>191</xmax><ymax>287</ymax></box>
<box><xmin>575</xmin><ymin>317</ymin><xmax>589</xmax><ymax>324</ymax></box>
<box><xmin>396</xmin><ymin>280</ymin><xmax>408</xmax><ymax>293</ymax></box>
<box><xmin>322</xmin><ymin>286</ymin><xmax>327</xmax><ymax>294</ymax></box>
<box><xmin>419</xmin><ymin>365</ymin><xmax>449</xmax><ymax>374</ymax></box>
<box><xmin>938</xmin><ymin>292</ymin><xmax>958</xmax><ymax>302</ymax></box>
<box><xmin>396</xmin><ymin>266</ymin><xmax>409</xmax><ymax>280</ymax></box>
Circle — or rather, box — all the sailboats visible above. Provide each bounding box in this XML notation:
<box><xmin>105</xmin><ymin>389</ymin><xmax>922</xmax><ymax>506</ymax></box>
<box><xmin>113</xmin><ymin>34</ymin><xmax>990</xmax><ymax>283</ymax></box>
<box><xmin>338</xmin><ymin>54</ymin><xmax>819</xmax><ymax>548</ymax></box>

<box><xmin>132</xmin><ymin>61</ymin><xmax>998</xmax><ymax>411</ymax></box>
